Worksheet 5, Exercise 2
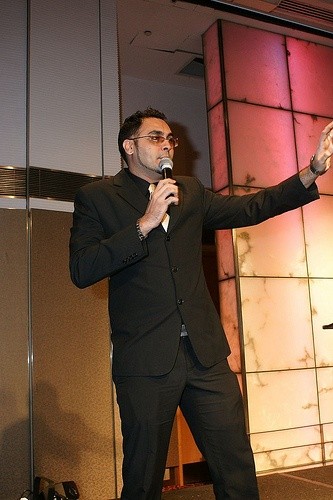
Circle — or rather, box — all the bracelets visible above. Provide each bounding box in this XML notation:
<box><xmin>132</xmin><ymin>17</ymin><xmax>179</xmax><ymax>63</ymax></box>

<box><xmin>309</xmin><ymin>155</ymin><xmax>328</xmax><ymax>177</ymax></box>
<box><xmin>136</xmin><ymin>218</ymin><xmax>149</xmax><ymax>242</ymax></box>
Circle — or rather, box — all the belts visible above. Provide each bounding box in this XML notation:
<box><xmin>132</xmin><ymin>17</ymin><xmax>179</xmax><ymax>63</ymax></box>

<box><xmin>180</xmin><ymin>325</ymin><xmax>188</xmax><ymax>337</ymax></box>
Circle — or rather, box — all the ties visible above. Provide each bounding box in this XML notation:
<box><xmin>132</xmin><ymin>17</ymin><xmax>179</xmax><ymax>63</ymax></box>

<box><xmin>148</xmin><ymin>184</ymin><xmax>171</xmax><ymax>232</ymax></box>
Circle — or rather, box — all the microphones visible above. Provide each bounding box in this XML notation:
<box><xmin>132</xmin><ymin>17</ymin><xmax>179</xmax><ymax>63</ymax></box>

<box><xmin>158</xmin><ymin>158</ymin><xmax>174</xmax><ymax>199</ymax></box>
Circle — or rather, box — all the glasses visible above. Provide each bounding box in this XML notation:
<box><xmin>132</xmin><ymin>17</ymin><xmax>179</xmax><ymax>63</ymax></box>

<box><xmin>132</xmin><ymin>135</ymin><xmax>179</xmax><ymax>148</ymax></box>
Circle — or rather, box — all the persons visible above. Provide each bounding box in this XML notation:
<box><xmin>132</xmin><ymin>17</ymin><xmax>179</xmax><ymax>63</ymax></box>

<box><xmin>68</xmin><ymin>108</ymin><xmax>333</xmax><ymax>500</ymax></box>
